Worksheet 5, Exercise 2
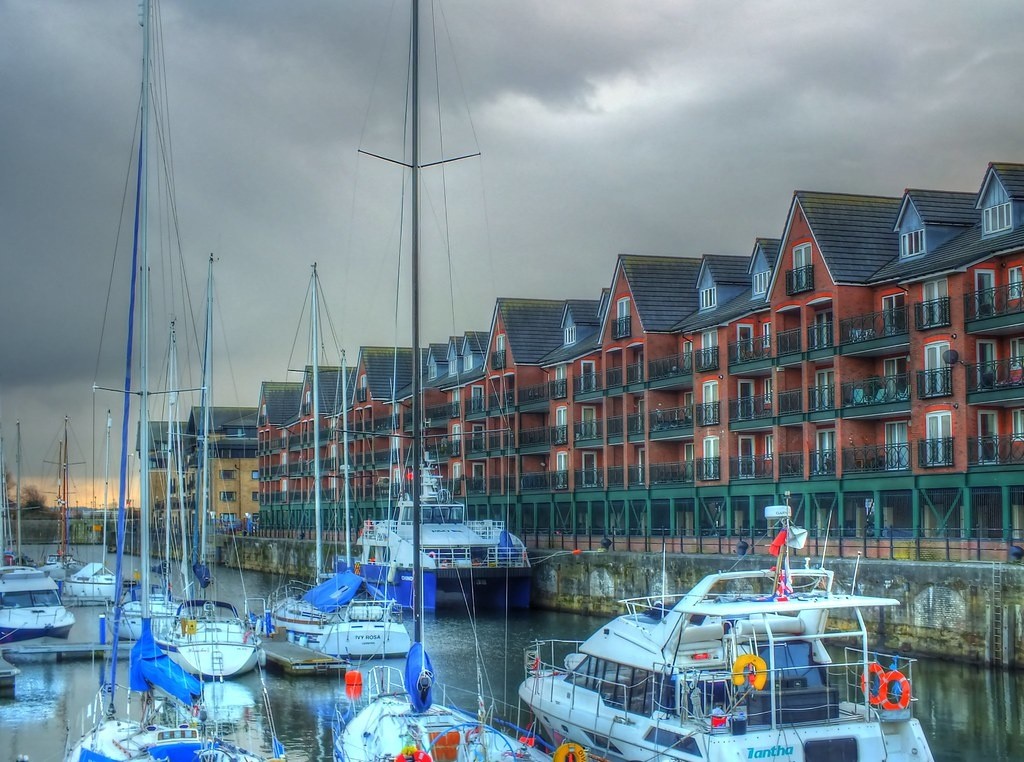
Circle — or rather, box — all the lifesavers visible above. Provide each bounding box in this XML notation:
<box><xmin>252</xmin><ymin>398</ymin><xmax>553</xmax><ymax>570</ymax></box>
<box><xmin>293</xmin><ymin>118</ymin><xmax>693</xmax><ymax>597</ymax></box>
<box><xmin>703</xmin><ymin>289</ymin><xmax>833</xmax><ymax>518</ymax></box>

<box><xmin>861</xmin><ymin>664</ymin><xmax>910</xmax><ymax>711</ymax></box>
<box><xmin>732</xmin><ymin>654</ymin><xmax>767</xmax><ymax>690</ymax></box>
<box><xmin>553</xmin><ymin>743</ymin><xmax>587</xmax><ymax>762</ymax></box>
<box><xmin>427</xmin><ymin>551</ymin><xmax>436</xmax><ymax>559</ymax></box>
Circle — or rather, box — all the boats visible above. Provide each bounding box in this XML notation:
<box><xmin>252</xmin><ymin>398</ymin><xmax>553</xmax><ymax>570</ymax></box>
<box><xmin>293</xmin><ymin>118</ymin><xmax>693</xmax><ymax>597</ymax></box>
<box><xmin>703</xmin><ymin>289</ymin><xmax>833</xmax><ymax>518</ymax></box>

<box><xmin>3</xmin><ymin>0</ymin><xmax>935</xmax><ymax>760</ymax></box>
